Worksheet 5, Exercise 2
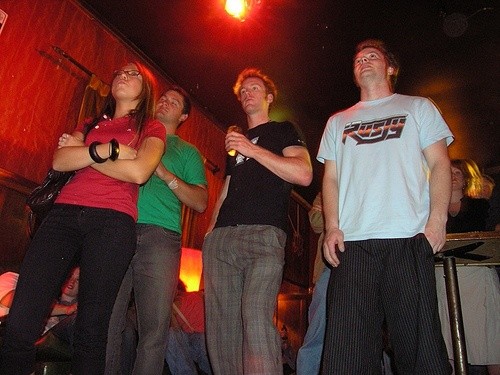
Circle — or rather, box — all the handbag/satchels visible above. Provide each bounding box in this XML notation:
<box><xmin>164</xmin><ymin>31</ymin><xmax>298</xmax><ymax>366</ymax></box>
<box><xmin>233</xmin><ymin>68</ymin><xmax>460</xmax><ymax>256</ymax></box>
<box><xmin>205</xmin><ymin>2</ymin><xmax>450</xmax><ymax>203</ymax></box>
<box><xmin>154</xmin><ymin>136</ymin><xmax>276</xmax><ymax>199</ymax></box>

<box><xmin>26</xmin><ymin>169</ymin><xmax>77</xmax><ymax>212</ymax></box>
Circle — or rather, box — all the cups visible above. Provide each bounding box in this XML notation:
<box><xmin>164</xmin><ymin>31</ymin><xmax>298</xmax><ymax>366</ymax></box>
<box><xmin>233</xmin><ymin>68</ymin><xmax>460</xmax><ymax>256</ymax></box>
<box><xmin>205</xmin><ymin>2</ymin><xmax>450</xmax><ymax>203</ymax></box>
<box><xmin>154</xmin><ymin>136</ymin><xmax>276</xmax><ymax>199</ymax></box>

<box><xmin>227</xmin><ymin>126</ymin><xmax>243</xmax><ymax>157</ymax></box>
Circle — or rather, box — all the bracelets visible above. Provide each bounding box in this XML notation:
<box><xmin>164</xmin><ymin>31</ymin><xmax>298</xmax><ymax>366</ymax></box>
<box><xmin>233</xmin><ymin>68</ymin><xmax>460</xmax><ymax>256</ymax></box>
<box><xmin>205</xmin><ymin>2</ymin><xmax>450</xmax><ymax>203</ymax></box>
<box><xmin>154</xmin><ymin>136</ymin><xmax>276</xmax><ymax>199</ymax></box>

<box><xmin>168</xmin><ymin>177</ymin><xmax>178</xmax><ymax>190</ymax></box>
<box><xmin>88</xmin><ymin>141</ymin><xmax>108</xmax><ymax>164</ymax></box>
<box><xmin>107</xmin><ymin>138</ymin><xmax>121</xmax><ymax>162</ymax></box>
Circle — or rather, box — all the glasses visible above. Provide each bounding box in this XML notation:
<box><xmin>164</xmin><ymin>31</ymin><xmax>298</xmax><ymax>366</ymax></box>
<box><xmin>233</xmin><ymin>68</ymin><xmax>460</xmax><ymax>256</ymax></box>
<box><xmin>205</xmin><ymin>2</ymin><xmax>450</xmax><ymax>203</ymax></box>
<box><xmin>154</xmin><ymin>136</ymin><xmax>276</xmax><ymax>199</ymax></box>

<box><xmin>114</xmin><ymin>69</ymin><xmax>140</xmax><ymax>76</ymax></box>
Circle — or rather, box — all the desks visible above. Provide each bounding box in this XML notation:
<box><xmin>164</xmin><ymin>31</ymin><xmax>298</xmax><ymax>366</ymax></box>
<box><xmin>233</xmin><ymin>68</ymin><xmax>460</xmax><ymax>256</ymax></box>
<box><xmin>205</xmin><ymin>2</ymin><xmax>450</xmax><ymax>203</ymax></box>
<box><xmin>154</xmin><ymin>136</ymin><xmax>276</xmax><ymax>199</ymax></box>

<box><xmin>437</xmin><ymin>229</ymin><xmax>500</xmax><ymax>375</ymax></box>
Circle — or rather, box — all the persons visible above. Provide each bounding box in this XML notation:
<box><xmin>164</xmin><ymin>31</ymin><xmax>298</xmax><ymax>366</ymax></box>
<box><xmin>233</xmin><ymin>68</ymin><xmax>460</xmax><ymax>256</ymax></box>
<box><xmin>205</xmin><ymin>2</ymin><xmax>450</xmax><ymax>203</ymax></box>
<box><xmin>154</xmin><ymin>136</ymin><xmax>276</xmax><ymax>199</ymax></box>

<box><xmin>0</xmin><ymin>193</ymin><xmax>212</xmax><ymax>375</ymax></box>
<box><xmin>317</xmin><ymin>38</ymin><xmax>456</xmax><ymax>375</ymax></box>
<box><xmin>1</xmin><ymin>53</ymin><xmax>169</xmax><ymax>375</ymax></box>
<box><xmin>438</xmin><ymin>159</ymin><xmax>498</xmax><ymax>235</ymax></box>
<box><xmin>103</xmin><ymin>86</ymin><xmax>208</xmax><ymax>375</ymax></box>
<box><xmin>295</xmin><ymin>188</ymin><xmax>333</xmax><ymax>374</ymax></box>
<box><xmin>201</xmin><ymin>66</ymin><xmax>313</xmax><ymax>375</ymax></box>
